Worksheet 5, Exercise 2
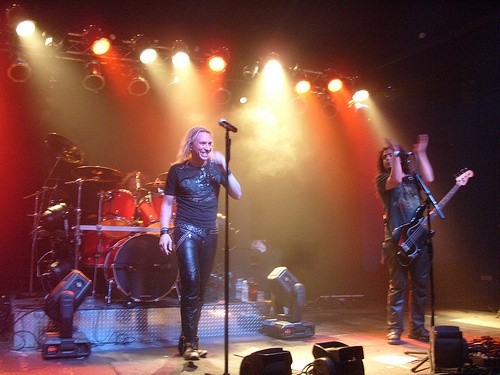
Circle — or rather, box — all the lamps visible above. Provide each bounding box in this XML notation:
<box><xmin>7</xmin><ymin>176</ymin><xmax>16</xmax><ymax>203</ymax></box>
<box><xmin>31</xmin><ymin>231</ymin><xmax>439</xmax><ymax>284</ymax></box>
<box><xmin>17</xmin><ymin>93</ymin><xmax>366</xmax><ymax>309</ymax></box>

<box><xmin>210</xmin><ymin>78</ymin><xmax>230</xmax><ymax>104</ymax></box>
<box><xmin>242</xmin><ymin>51</ymin><xmax>371</xmax><ymax>118</ymax></box>
<box><xmin>127</xmin><ymin>66</ymin><xmax>149</xmax><ymax>96</ymax></box>
<box><xmin>239</xmin><ymin>348</ymin><xmax>293</xmax><ymax>375</ymax></box>
<box><xmin>208</xmin><ymin>42</ymin><xmax>229</xmax><ymax>71</ymax></box>
<box><xmin>82</xmin><ymin>60</ymin><xmax>107</xmax><ymax>95</ymax></box>
<box><xmin>168</xmin><ymin>36</ymin><xmax>189</xmax><ymax>60</ymax></box>
<box><xmin>428</xmin><ymin>323</ymin><xmax>471</xmax><ymax>372</ymax></box>
<box><xmin>314</xmin><ymin>337</ymin><xmax>365</xmax><ymax>375</ymax></box>
<box><xmin>262</xmin><ymin>265</ymin><xmax>315</xmax><ymax>339</ymax></box>
<box><xmin>130</xmin><ymin>34</ymin><xmax>158</xmax><ymax>65</ymax></box>
<box><xmin>8</xmin><ymin>48</ymin><xmax>30</xmax><ymax>84</ymax></box>
<box><xmin>81</xmin><ymin>23</ymin><xmax>112</xmax><ymax>57</ymax></box>
<box><xmin>3</xmin><ymin>3</ymin><xmax>32</xmax><ymax>32</ymax></box>
<box><xmin>42</xmin><ymin>270</ymin><xmax>92</xmax><ymax>358</ymax></box>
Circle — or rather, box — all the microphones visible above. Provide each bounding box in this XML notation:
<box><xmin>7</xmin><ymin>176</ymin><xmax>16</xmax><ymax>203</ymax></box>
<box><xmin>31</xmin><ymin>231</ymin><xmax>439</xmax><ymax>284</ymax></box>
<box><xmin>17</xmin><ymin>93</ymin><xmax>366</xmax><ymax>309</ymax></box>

<box><xmin>218</xmin><ymin>118</ymin><xmax>238</xmax><ymax>133</ymax></box>
<box><xmin>393</xmin><ymin>151</ymin><xmax>415</xmax><ymax>156</ymax></box>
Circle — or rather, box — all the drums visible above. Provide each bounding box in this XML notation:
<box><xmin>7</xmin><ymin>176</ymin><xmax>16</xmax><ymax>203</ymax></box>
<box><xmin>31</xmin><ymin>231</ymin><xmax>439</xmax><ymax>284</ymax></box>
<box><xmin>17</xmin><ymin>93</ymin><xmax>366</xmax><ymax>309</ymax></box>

<box><xmin>136</xmin><ymin>192</ymin><xmax>174</xmax><ymax>227</ymax></box>
<box><xmin>98</xmin><ymin>189</ymin><xmax>137</xmax><ymax>239</ymax></box>
<box><xmin>173</xmin><ymin>201</ymin><xmax>177</xmax><ymax>220</ymax></box>
<box><xmin>80</xmin><ymin>231</ymin><xmax>119</xmax><ymax>269</ymax></box>
<box><xmin>103</xmin><ymin>233</ymin><xmax>181</xmax><ymax>303</ymax></box>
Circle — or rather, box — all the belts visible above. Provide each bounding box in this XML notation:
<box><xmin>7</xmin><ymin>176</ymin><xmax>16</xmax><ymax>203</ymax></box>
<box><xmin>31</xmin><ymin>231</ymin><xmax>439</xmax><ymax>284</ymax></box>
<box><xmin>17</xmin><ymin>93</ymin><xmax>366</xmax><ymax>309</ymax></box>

<box><xmin>177</xmin><ymin>223</ymin><xmax>217</xmax><ymax>238</ymax></box>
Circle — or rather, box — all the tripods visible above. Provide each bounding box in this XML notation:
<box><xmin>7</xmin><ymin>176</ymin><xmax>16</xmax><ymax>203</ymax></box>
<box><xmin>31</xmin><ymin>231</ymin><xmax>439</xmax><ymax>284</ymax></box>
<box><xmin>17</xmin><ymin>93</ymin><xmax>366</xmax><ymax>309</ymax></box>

<box><xmin>401</xmin><ymin>155</ymin><xmax>445</xmax><ymax>372</ymax></box>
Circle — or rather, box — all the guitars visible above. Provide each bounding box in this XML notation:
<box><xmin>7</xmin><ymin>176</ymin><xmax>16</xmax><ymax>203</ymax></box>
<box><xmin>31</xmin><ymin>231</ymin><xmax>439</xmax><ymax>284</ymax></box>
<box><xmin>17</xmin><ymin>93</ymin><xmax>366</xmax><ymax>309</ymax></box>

<box><xmin>389</xmin><ymin>168</ymin><xmax>474</xmax><ymax>267</ymax></box>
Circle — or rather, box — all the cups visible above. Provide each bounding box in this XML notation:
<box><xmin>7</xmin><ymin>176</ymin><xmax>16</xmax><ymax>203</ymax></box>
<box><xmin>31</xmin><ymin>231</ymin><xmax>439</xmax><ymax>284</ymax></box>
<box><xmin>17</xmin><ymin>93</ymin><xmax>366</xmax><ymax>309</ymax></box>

<box><xmin>256</xmin><ymin>291</ymin><xmax>264</xmax><ymax>302</ymax></box>
<box><xmin>282</xmin><ymin>306</ymin><xmax>288</xmax><ymax>315</ymax></box>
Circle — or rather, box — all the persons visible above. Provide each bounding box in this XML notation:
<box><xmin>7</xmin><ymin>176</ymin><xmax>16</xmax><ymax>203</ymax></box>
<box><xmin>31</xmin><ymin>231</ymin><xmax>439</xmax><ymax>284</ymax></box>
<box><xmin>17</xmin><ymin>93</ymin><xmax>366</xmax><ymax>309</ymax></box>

<box><xmin>375</xmin><ymin>134</ymin><xmax>434</xmax><ymax>344</ymax></box>
<box><xmin>157</xmin><ymin>127</ymin><xmax>240</xmax><ymax>360</ymax></box>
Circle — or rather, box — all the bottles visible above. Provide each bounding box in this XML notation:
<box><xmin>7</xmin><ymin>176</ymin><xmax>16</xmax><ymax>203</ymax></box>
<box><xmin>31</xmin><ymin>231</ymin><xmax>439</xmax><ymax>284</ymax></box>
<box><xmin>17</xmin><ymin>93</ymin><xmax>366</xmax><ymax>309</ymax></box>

<box><xmin>241</xmin><ymin>280</ymin><xmax>249</xmax><ymax>302</ymax></box>
<box><xmin>235</xmin><ymin>278</ymin><xmax>242</xmax><ymax>299</ymax></box>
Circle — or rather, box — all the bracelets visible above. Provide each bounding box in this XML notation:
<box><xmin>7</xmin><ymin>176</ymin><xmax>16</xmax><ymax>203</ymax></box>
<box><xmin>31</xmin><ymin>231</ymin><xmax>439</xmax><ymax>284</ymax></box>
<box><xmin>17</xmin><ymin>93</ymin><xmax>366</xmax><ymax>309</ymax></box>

<box><xmin>223</xmin><ymin>170</ymin><xmax>231</xmax><ymax>176</ymax></box>
<box><xmin>160</xmin><ymin>227</ymin><xmax>169</xmax><ymax>235</ymax></box>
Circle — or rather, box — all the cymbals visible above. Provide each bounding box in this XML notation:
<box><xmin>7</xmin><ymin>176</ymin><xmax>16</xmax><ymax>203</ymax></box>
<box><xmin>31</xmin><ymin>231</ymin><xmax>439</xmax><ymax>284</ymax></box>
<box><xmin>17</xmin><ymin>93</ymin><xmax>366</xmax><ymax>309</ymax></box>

<box><xmin>70</xmin><ymin>166</ymin><xmax>125</xmax><ymax>182</ymax></box>
<box><xmin>144</xmin><ymin>181</ymin><xmax>166</xmax><ymax>188</ymax></box>
<box><xmin>47</xmin><ymin>133</ymin><xmax>81</xmax><ymax>164</ymax></box>
<box><xmin>159</xmin><ymin>172</ymin><xmax>168</xmax><ymax>179</ymax></box>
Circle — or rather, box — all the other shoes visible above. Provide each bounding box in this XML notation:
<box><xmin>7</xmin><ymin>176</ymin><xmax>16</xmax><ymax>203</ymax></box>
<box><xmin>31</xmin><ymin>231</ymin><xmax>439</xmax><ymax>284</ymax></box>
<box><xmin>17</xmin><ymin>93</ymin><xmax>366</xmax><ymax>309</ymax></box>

<box><xmin>408</xmin><ymin>325</ymin><xmax>429</xmax><ymax>340</ymax></box>
<box><xmin>182</xmin><ymin>347</ymin><xmax>199</xmax><ymax>360</ymax></box>
<box><xmin>387</xmin><ymin>331</ymin><xmax>401</xmax><ymax>343</ymax></box>
<box><xmin>197</xmin><ymin>349</ymin><xmax>208</xmax><ymax>356</ymax></box>
